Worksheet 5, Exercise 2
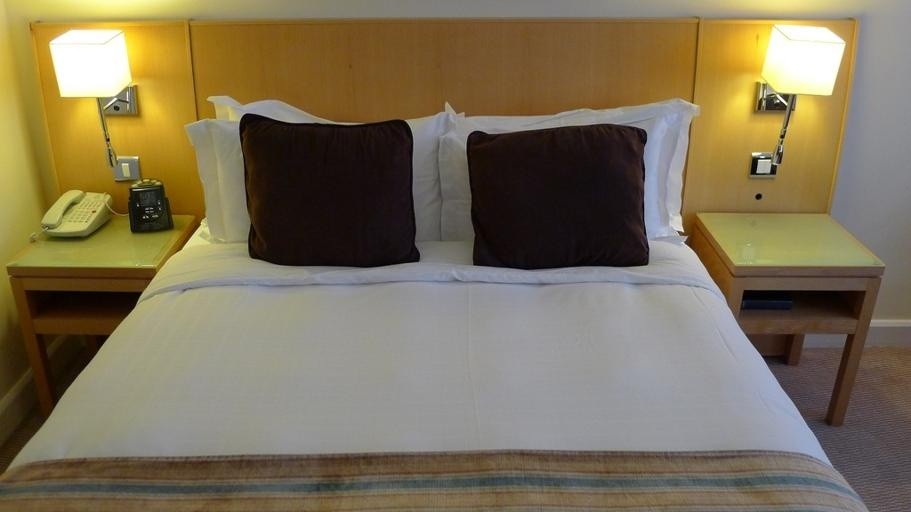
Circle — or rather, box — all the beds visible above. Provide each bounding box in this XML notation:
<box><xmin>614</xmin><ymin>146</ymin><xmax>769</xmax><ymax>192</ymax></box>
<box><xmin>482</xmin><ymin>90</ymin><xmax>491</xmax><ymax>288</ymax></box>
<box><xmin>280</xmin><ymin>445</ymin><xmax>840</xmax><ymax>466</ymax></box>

<box><xmin>0</xmin><ymin>21</ymin><xmax>869</xmax><ymax>512</ymax></box>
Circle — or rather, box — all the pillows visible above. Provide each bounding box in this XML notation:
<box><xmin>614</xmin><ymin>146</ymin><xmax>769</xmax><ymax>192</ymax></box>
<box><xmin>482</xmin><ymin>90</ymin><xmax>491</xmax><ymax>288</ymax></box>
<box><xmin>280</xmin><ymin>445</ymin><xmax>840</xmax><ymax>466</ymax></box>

<box><xmin>239</xmin><ymin>114</ymin><xmax>420</xmax><ymax>268</ymax></box>
<box><xmin>184</xmin><ymin>112</ymin><xmax>447</xmax><ymax>246</ymax></box>
<box><xmin>441</xmin><ymin>99</ymin><xmax>701</xmax><ymax>243</ymax></box>
<box><xmin>466</xmin><ymin>124</ymin><xmax>649</xmax><ymax>270</ymax></box>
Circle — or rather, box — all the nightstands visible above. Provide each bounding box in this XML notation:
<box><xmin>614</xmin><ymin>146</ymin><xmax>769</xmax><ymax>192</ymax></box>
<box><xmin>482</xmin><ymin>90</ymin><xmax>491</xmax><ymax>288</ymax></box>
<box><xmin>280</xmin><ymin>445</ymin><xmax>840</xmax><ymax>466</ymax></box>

<box><xmin>692</xmin><ymin>212</ymin><xmax>886</xmax><ymax>426</ymax></box>
<box><xmin>6</xmin><ymin>214</ymin><xmax>197</xmax><ymax>417</ymax></box>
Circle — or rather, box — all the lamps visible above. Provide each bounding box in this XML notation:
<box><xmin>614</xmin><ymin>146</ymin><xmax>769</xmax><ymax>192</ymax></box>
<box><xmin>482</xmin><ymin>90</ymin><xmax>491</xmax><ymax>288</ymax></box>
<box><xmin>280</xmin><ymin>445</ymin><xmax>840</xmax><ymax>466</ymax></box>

<box><xmin>755</xmin><ymin>24</ymin><xmax>845</xmax><ymax>165</ymax></box>
<box><xmin>49</xmin><ymin>29</ymin><xmax>138</xmax><ymax>167</ymax></box>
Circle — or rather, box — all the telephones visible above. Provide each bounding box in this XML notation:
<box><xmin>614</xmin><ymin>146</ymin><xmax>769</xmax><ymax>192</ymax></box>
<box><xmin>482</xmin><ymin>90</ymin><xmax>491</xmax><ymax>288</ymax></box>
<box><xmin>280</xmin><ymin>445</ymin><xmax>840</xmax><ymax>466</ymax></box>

<box><xmin>28</xmin><ymin>188</ymin><xmax>113</xmax><ymax>243</ymax></box>
